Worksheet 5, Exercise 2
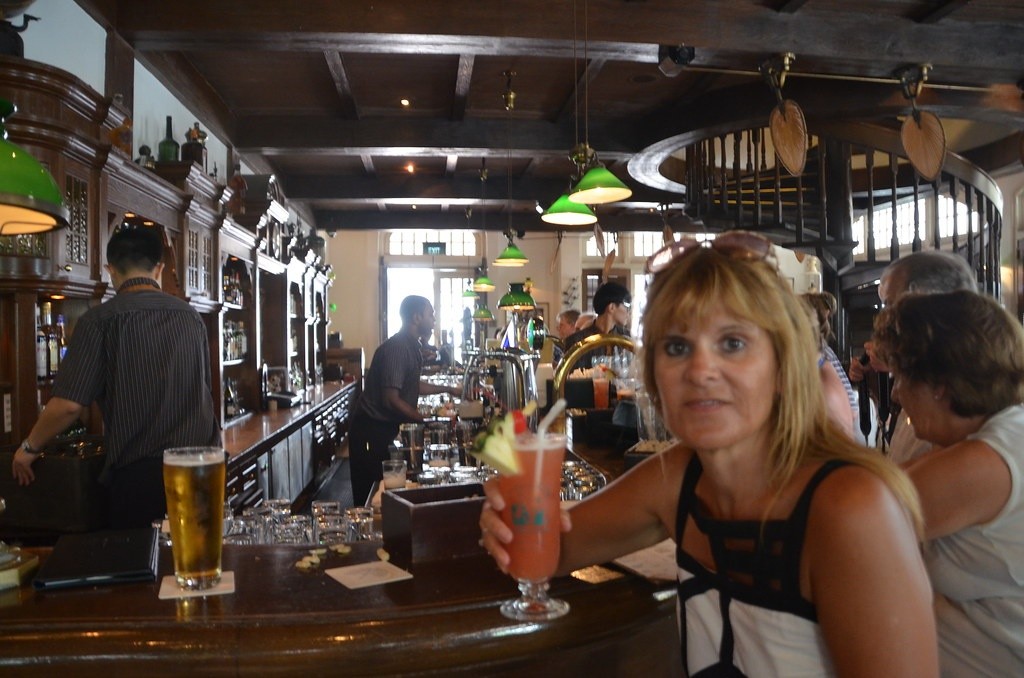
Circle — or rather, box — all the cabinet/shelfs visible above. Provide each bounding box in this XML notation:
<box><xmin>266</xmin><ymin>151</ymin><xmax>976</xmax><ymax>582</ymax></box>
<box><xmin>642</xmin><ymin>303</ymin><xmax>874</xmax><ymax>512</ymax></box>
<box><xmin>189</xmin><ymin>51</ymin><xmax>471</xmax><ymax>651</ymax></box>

<box><xmin>2</xmin><ymin>58</ymin><xmax>359</xmax><ymax>515</ymax></box>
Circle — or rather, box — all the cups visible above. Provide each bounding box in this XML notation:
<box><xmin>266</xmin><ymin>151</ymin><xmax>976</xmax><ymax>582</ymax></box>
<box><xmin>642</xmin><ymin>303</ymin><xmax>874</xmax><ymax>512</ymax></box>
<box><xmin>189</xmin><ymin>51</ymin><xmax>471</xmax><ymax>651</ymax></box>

<box><xmin>163</xmin><ymin>447</ymin><xmax>224</xmax><ymax>590</ymax></box>
<box><xmin>590</xmin><ymin>346</ymin><xmax>670</xmax><ymax>449</ymax></box>
<box><xmin>490</xmin><ymin>434</ymin><xmax>571</xmax><ymax>621</ymax></box>
<box><xmin>221</xmin><ymin>428</ymin><xmax>606</xmax><ymax>547</ymax></box>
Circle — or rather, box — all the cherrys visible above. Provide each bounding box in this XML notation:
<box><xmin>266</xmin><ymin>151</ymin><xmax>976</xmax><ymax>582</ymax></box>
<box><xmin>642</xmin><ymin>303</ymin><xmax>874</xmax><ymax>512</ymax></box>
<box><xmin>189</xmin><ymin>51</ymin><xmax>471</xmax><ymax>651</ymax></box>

<box><xmin>512</xmin><ymin>409</ymin><xmax>525</xmax><ymax>434</ymax></box>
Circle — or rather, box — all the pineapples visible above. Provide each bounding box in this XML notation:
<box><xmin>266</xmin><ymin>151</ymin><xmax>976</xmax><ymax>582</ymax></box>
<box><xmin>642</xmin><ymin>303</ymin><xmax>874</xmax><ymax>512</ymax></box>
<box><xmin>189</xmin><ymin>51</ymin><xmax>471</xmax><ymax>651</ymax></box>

<box><xmin>468</xmin><ymin>411</ymin><xmax>518</xmax><ymax>478</ymax></box>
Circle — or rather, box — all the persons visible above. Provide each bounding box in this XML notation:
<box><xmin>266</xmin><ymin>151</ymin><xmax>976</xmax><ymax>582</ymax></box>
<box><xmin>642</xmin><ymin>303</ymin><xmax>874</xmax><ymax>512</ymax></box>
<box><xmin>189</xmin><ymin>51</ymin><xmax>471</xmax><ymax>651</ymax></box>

<box><xmin>480</xmin><ymin>243</ymin><xmax>938</xmax><ymax>678</ymax></box>
<box><xmin>11</xmin><ymin>229</ymin><xmax>214</xmax><ymax>529</ymax></box>
<box><xmin>347</xmin><ymin>296</ymin><xmax>462</xmax><ymax>508</ymax></box>
<box><xmin>552</xmin><ymin>282</ymin><xmax>632</xmax><ymax>408</ymax></box>
<box><xmin>871</xmin><ymin>289</ymin><xmax>1024</xmax><ymax>678</ymax></box>
<box><xmin>849</xmin><ymin>248</ymin><xmax>976</xmax><ymax>462</ymax></box>
<box><xmin>800</xmin><ymin>291</ymin><xmax>865</xmax><ymax>446</ymax></box>
<box><xmin>419</xmin><ymin>329</ymin><xmax>437</xmax><ymax>362</ymax></box>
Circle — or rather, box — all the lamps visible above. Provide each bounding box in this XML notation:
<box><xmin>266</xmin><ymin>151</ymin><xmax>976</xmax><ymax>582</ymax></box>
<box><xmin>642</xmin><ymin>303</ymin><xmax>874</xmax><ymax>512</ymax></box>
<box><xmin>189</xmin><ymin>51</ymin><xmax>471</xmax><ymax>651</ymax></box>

<box><xmin>0</xmin><ymin>100</ymin><xmax>75</xmax><ymax>236</ymax></box>
<box><xmin>570</xmin><ymin>0</ymin><xmax>634</xmax><ymax>205</ymax></box>
<box><xmin>541</xmin><ymin>1</ymin><xmax>599</xmax><ymax>224</ymax></box>
<box><xmin>464</xmin><ymin>68</ymin><xmax>536</xmax><ymax>319</ymax></box>
<box><xmin>657</xmin><ymin>45</ymin><xmax>697</xmax><ymax>76</ymax></box>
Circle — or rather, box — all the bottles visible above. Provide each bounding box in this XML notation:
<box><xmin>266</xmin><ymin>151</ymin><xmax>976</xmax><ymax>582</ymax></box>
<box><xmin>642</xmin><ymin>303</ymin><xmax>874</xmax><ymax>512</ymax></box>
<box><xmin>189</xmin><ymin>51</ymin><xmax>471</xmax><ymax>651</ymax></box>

<box><xmin>158</xmin><ymin>116</ymin><xmax>179</xmax><ymax>162</ymax></box>
<box><xmin>223</xmin><ymin>267</ymin><xmax>250</xmax><ymax>421</ymax></box>
<box><xmin>32</xmin><ymin>300</ymin><xmax>91</xmax><ymax>436</ymax></box>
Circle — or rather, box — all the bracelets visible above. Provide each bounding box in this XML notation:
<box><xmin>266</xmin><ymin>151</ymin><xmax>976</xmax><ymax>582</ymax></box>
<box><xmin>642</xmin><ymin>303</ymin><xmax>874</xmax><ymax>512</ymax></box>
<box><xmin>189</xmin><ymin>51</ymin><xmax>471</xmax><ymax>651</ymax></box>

<box><xmin>22</xmin><ymin>439</ymin><xmax>40</xmax><ymax>454</ymax></box>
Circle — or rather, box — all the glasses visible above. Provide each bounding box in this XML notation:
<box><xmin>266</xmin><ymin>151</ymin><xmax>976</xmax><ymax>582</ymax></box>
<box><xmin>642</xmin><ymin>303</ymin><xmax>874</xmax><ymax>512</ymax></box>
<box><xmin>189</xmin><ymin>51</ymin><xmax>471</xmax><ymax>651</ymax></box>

<box><xmin>643</xmin><ymin>230</ymin><xmax>774</xmax><ymax>274</ymax></box>
